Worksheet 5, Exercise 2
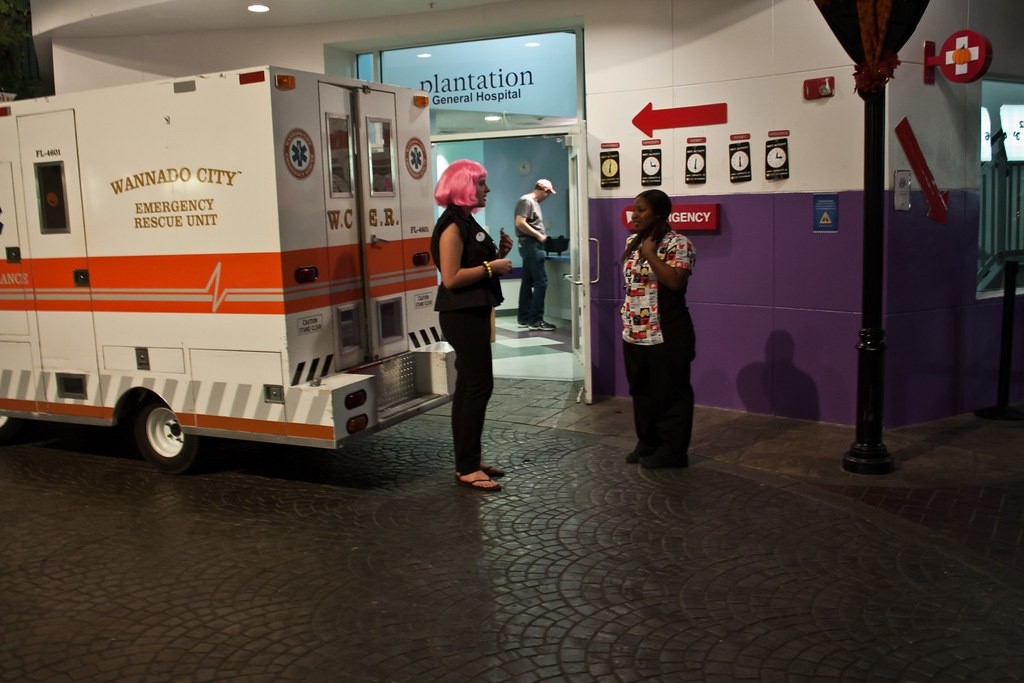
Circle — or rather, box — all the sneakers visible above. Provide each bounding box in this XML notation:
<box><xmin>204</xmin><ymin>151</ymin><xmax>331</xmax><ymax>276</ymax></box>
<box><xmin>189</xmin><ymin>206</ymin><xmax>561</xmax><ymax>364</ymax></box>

<box><xmin>528</xmin><ymin>320</ymin><xmax>556</xmax><ymax>331</ymax></box>
<box><xmin>517</xmin><ymin>320</ymin><xmax>530</xmax><ymax>328</ymax></box>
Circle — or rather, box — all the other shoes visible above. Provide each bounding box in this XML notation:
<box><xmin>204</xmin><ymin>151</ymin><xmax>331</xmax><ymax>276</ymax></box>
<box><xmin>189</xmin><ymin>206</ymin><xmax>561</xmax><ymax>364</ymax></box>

<box><xmin>627</xmin><ymin>446</ymin><xmax>654</xmax><ymax>462</ymax></box>
<box><xmin>643</xmin><ymin>449</ymin><xmax>689</xmax><ymax>469</ymax></box>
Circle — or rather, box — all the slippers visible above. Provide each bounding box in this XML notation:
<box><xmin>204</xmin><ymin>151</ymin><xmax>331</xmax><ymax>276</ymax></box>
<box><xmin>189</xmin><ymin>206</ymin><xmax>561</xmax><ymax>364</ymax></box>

<box><xmin>458</xmin><ymin>476</ymin><xmax>501</xmax><ymax>492</ymax></box>
<box><xmin>456</xmin><ymin>466</ymin><xmax>505</xmax><ymax>477</ymax></box>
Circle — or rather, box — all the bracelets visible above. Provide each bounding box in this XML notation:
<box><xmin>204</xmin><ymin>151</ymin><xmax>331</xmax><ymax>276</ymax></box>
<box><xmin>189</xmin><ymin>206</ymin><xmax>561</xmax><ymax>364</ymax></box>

<box><xmin>484</xmin><ymin>261</ymin><xmax>493</xmax><ymax>279</ymax></box>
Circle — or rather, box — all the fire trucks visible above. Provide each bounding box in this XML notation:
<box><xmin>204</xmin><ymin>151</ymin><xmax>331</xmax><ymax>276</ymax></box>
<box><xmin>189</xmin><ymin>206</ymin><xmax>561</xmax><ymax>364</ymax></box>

<box><xmin>0</xmin><ymin>66</ymin><xmax>455</xmax><ymax>477</ymax></box>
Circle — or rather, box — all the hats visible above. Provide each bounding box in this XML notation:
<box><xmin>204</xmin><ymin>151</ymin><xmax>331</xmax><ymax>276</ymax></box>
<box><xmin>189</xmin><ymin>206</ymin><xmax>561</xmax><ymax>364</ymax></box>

<box><xmin>536</xmin><ymin>178</ymin><xmax>556</xmax><ymax>194</ymax></box>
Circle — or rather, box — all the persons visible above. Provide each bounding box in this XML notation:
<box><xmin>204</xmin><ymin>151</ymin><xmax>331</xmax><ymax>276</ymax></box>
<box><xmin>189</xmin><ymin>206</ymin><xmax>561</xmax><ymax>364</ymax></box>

<box><xmin>514</xmin><ymin>178</ymin><xmax>557</xmax><ymax>331</ymax></box>
<box><xmin>429</xmin><ymin>159</ymin><xmax>514</xmax><ymax>492</ymax></box>
<box><xmin>620</xmin><ymin>189</ymin><xmax>699</xmax><ymax>468</ymax></box>
